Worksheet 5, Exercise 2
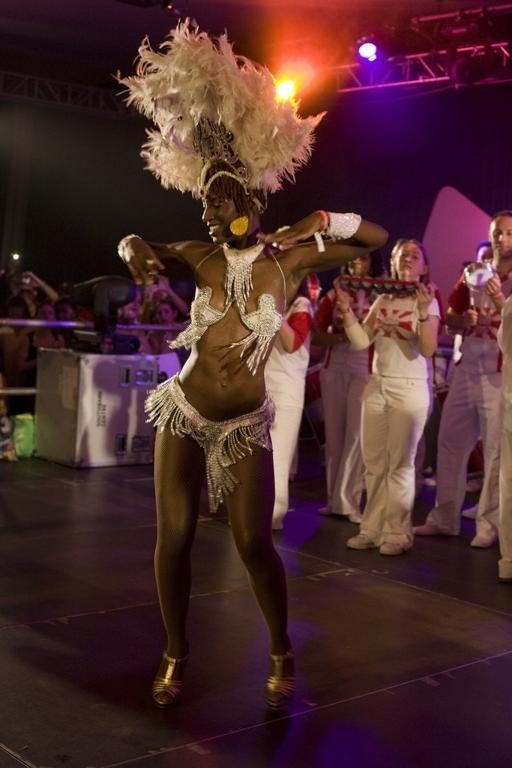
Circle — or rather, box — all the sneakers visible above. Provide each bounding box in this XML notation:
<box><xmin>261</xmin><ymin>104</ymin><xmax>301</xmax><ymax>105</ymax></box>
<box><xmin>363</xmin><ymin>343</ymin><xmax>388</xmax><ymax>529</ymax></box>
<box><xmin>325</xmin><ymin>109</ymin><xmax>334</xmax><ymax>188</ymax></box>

<box><xmin>319</xmin><ymin>502</ymin><xmax>494</xmax><ymax>555</ymax></box>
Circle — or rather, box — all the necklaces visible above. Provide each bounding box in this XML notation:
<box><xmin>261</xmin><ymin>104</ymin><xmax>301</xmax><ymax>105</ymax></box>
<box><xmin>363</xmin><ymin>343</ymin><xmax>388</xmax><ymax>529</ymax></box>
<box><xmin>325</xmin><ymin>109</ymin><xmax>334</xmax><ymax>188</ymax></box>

<box><xmin>222</xmin><ymin>240</ymin><xmax>267</xmax><ymax>312</ymax></box>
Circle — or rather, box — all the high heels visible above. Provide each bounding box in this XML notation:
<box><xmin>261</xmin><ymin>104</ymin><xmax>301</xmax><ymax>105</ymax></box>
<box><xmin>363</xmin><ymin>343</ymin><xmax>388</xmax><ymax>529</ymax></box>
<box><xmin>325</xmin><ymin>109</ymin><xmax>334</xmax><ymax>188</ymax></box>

<box><xmin>264</xmin><ymin>652</ymin><xmax>296</xmax><ymax>712</ymax></box>
<box><xmin>152</xmin><ymin>650</ymin><xmax>185</xmax><ymax>705</ymax></box>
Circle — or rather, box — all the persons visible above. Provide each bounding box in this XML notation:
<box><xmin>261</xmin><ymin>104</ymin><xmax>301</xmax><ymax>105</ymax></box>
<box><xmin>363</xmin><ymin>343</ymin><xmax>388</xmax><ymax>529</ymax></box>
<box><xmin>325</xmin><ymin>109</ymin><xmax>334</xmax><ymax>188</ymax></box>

<box><xmin>0</xmin><ymin>258</ymin><xmax>90</xmax><ymax>413</ymax></box>
<box><xmin>264</xmin><ymin>212</ymin><xmax>512</xmax><ymax>581</ymax></box>
<box><xmin>116</xmin><ymin>163</ymin><xmax>388</xmax><ymax>709</ymax></box>
<box><xmin>117</xmin><ymin>281</ymin><xmax>188</xmax><ymax>355</ymax></box>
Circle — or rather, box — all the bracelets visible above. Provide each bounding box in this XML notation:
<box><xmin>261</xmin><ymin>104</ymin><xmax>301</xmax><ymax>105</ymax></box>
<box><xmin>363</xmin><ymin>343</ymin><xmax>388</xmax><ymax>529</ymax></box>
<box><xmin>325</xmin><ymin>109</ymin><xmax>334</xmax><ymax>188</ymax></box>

<box><xmin>116</xmin><ymin>233</ymin><xmax>142</xmax><ymax>264</ymax></box>
